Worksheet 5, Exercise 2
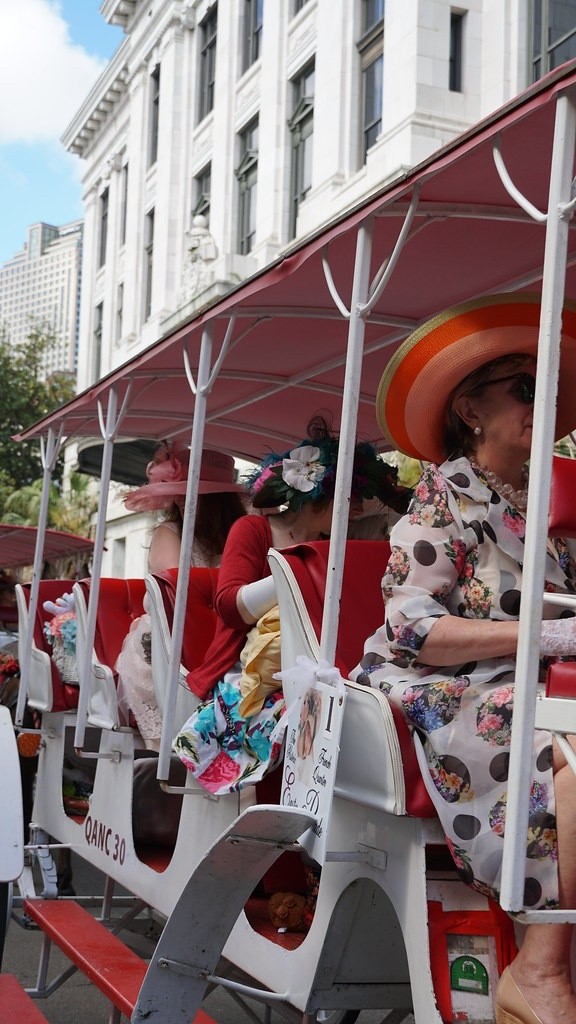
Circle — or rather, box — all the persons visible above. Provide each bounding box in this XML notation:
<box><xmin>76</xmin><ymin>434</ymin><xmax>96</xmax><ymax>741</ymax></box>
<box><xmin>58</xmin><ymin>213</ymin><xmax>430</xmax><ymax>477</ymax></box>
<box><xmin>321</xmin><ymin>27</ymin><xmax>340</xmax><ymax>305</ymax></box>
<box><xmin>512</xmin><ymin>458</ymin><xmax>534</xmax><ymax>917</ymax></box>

<box><xmin>0</xmin><ymin>575</ymin><xmax>19</xmax><ymax>631</ymax></box>
<box><xmin>172</xmin><ymin>436</ymin><xmax>375</xmax><ymax>797</ymax></box>
<box><xmin>113</xmin><ymin>447</ymin><xmax>248</xmax><ymax>741</ymax></box>
<box><xmin>347</xmin><ymin>288</ymin><xmax>576</xmax><ymax>1024</ymax></box>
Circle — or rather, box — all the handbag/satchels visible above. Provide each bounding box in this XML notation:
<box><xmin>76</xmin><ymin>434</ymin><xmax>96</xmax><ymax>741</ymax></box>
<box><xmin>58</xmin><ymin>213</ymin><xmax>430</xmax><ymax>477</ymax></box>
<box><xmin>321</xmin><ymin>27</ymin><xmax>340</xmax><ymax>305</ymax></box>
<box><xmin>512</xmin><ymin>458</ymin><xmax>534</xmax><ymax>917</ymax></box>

<box><xmin>43</xmin><ymin>592</ymin><xmax>81</xmax><ymax>684</ymax></box>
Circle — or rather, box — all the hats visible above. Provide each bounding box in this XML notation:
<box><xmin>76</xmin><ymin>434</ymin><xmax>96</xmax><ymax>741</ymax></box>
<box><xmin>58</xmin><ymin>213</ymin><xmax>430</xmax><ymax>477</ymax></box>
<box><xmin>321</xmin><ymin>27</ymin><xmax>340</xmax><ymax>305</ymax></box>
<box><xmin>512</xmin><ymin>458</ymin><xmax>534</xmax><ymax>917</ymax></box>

<box><xmin>248</xmin><ymin>408</ymin><xmax>415</xmax><ymax>516</ymax></box>
<box><xmin>376</xmin><ymin>291</ymin><xmax>576</xmax><ymax>466</ymax></box>
<box><xmin>122</xmin><ymin>437</ymin><xmax>245</xmax><ymax>511</ymax></box>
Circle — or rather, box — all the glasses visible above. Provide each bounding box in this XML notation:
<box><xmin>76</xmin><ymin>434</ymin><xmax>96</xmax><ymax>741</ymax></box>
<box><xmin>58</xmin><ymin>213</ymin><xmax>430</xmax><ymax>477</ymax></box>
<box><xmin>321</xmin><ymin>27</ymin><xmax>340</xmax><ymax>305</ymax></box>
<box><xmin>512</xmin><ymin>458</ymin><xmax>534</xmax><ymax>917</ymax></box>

<box><xmin>471</xmin><ymin>371</ymin><xmax>535</xmax><ymax>404</ymax></box>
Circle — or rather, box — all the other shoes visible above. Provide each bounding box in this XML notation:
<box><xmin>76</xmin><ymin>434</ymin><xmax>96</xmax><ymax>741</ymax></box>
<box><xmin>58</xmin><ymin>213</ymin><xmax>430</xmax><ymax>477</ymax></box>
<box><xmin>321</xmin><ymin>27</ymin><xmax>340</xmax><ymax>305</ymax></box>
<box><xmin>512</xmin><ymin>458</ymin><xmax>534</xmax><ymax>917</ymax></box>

<box><xmin>495</xmin><ymin>964</ymin><xmax>542</xmax><ymax>1024</ymax></box>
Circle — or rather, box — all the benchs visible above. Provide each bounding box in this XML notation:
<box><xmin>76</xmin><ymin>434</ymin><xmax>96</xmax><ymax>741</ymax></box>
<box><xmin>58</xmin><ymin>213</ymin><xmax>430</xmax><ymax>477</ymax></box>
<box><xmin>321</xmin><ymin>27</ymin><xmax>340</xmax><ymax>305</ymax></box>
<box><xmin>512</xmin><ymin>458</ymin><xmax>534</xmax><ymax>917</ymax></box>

<box><xmin>268</xmin><ymin>538</ymin><xmax>438</xmax><ymax>819</ymax></box>
<box><xmin>141</xmin><ymin>565</ymin><xmax>258</xmax><ymax>811</ymax></box>
<box><xmin>73</xmin><ymin>575</ymin><xmax>147</xmax><ymax>734</ymax></box>
<box><xmin>13</xmin><ymin>577</ymin><xmax>78</xmax><ymax>714</ymax></box>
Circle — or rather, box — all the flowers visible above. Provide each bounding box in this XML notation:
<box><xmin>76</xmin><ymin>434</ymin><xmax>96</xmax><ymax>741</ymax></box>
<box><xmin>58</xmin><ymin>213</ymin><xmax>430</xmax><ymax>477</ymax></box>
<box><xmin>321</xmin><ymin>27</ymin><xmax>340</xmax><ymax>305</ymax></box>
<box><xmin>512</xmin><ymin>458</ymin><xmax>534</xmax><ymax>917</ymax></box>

<box><xmin>281</xmin><ymin>445</ymin><xmax>327</xmax><ymax>493</ymax></box>
